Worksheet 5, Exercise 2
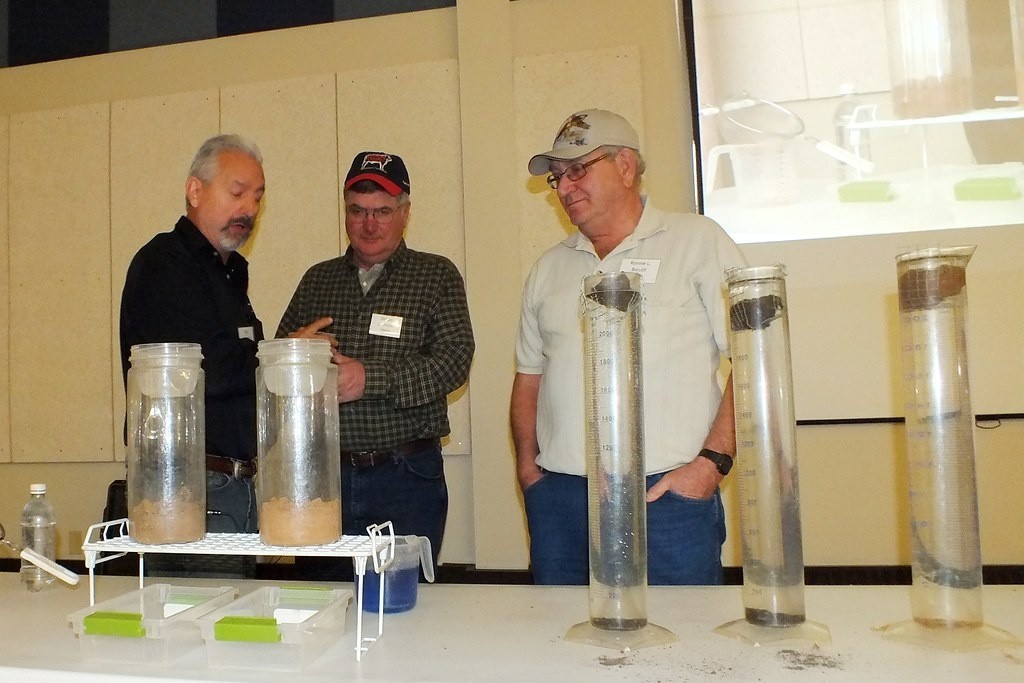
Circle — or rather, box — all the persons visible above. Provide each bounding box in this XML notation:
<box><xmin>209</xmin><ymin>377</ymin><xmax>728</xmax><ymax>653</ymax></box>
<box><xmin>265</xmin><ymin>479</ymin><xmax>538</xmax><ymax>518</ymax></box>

<box><xmin>272</xmin><ymin>150</ymin><xmax>474</xmax><ymax>591</ymax></box>
<box><xmin>505</xmin><ymin>107</ymin><xmax>764</xmax><ymax>587</ymax></box>
<box><xmin>121</xmin><ymin>132</ymin><xmax>345</xmax><ymax>579</ymax></box>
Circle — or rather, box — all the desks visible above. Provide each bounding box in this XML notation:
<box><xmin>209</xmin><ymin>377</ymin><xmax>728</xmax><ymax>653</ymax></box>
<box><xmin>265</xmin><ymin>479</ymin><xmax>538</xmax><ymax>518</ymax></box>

<box><xmin>0</xmin><ymin>571</ymin><xmax>1024</xmax><ymax>683</ymax></box>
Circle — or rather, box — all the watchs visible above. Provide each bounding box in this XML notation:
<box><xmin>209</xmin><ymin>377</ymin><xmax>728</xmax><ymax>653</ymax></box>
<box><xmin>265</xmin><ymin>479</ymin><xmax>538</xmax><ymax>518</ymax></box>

<box><xmin>698</xmin><ymin>447</ymin><xmax>734</xmax><ymax>477</ymax></box>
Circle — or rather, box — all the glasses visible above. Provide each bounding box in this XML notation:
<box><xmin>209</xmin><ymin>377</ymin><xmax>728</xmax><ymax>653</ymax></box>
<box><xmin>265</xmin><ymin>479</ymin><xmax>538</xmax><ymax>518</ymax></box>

<box><xmin>547</xmin><ymin>153</ymin><xmax>611</xmax><ymax>189</ymax></box>
<box><xmin>345</xmin><ymin>207</ymin><xmax>399</xmax><ymax>224</ymax></box>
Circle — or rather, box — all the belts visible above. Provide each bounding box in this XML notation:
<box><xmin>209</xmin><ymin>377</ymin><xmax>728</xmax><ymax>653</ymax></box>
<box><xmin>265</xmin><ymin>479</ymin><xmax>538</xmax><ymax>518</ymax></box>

<box><xmin>205</xmin><ymin>456</ymin><xmax>258</xmax><ymax>479</ymax></box>
<box><xmin>340</xmin><ymin>438</ymin><xmax>441</xmax><ymax>466</ymax></box>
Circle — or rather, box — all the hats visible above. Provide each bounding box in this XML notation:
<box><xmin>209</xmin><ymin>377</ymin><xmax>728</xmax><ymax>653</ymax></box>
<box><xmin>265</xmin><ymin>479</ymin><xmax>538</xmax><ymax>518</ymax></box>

<box><xmin>527</xmin><ymin>108</ymin><xmax>640</xmax><ymax>177</ymax></box>
<box><xmin>343</xmin><ymin>151</ymin><xmax>410</xmax><ymax>197</ymax></box>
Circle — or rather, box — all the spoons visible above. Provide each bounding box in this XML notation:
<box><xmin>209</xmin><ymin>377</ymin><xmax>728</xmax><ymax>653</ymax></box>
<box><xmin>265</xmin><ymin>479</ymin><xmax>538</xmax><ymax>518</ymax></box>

<box><xmin>0</xmin><ymin>522</ymin><xmax>79</xmax><ymax>585</ymax></box>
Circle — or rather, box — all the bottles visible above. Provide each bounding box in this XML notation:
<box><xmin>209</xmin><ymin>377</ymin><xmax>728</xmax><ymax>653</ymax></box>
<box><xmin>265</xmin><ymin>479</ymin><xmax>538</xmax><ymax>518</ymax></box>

<box><xmin>892</xmin><ymin>242</ymin><xmax>988</xmax><ymax>633</ymax></box>
<box><xmin>19</xmin><ymin>483</ymin><xmax>57</xmax><ymax>591</ymax></box>
<box><xmin>126</xmin><ymin>342</ymin><xmax>207</xmax><ymax>543</ymax></box>
<box><xmin>582</xmin><ymin>270</ymin><xmax>648</xmax><ymax>632</ymax></box>
<box><xmin>254</xmin><ymin>338</ymin><xmax>342</xmax><ymax>546</ymax></box>
<box><xmin>727</xmin><ymin>264</ymin><xmax>805</xmax><ymax>628</ymax></box>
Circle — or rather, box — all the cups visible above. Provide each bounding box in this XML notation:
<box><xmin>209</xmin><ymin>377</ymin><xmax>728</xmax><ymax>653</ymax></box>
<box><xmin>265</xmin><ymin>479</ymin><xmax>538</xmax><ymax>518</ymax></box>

<box><xmin>352</xmin><ymin>536</ymin><xmax>436</xmax><ymax>613</ymax></box>
